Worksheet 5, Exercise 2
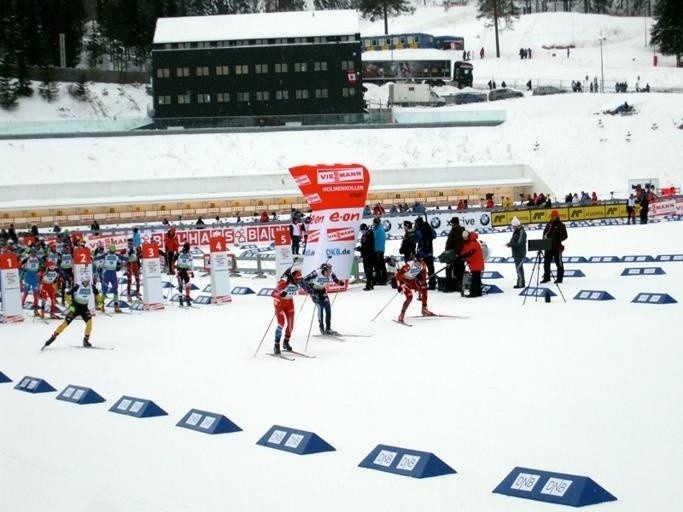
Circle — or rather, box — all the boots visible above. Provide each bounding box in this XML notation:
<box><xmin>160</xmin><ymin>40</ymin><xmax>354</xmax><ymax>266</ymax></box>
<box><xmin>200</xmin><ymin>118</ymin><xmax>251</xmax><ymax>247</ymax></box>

<box><xmin>83</xmin><ymin>338</ymin><xmax>92</xmax><ymax>346</ymax></box>
<box><xmin>274</xmin><ymin>339</ymin><xmax>280</xmax><ymax>354</ymax></box>
<box><xmin>283</xmin><ymin>339</ymin><xmax>293</xmax><ymax>350</ymax></box>
<box><xmin>46</xmin><ymin>336</ymin><xmax>56</xmax><ymax>345</ymax></box>
<box><xmin>422</xmin><ymin>308</ymin><xmax>434</xmax><ymax>316</ymax></box>
<box><xmin>114</xmin><ymin>304</ymin><xmax>121</xmax><ymax>312</ymax></box>
<box><xmin>399</xmin><ymin>313</ymin><xmax>404</xmax><ymax>321</ymax></box>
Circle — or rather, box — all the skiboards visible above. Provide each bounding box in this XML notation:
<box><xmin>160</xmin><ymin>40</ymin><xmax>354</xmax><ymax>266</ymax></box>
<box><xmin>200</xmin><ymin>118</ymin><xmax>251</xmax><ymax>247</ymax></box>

<box><xmin>392</xmin><ymin>315</ymin><xmax>468</xmax><ymax>327</ymax></box>
<box><xmin>176</xmin><ymin>305</ymin><xmax>201</xmax><ymax>310</ymax></box>
<box><xmin>102</xmin><ymin>311</ymin><xmax>134</xmax><ymax>317</ymax></box>
<box><xmin>310</xmin><ymin>335</ymin><xmax>373</xmax><ymax>342</ymax></box>
<box><xmin>267</xmin><ymin>349</ymin><xmax>317</xmax><ymax>361</ymax></box>
<box><xmin>41</xmin><ymin>345</ymin><xmax>114</xmax><ymax>350</ymax></box>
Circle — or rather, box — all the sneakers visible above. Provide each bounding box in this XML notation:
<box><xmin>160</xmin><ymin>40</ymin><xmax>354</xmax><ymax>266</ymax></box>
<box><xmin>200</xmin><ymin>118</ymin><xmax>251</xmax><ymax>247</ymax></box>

<box><xmin>321</xmin><ymin>327</ymin><xmax>338</xmax><ymax>335</ymax></box>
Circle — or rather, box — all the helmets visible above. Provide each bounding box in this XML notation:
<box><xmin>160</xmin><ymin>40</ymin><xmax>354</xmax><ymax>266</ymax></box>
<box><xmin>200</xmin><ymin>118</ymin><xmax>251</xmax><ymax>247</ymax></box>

<box><xmin>323</xmin><ymin>264</ymin><xmax>332</xmax><ymax>271</ymax></box>
<box><xmin>108</xmin><ymin>245</ymin><xmax>116</xmax><ymax>252</ymax></box>
<box><xmin>416</xmin><ymin>252</ymin><xmax>424</xmax><ymax>260</ymax></box>
<box><xmin>81</xmin><ymin>272</ymin><xmax>91</xmax><ymax>284</ymax></box>
<box><xmin>293</xmin><ymin>272</ymin><xmax>302</xmax><ymax>281</ymax></box>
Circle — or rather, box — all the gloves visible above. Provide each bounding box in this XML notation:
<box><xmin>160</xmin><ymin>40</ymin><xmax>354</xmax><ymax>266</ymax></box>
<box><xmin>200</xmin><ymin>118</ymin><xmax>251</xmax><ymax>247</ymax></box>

<box><xmin>280</xmin><ymin>291</ymin><xmax>287</xmax><ymax>297</ymax></box>
<box><xmin>338</xmin><ymin>280</ymin><xmax>344</xmax><ymax>286</ymax></box>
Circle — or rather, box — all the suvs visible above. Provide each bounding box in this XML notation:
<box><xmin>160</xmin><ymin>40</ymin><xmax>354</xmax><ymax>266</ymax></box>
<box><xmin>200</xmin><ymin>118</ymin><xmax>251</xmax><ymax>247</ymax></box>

<box><xmin>488</xmin><ymin>88</ymin><xmax>522</xmax><ymax>102</ymax></box>
<box><xmin>531</xmin><ymin>86</ymin><xmax>566</xmax><ymax>95</ymax></box>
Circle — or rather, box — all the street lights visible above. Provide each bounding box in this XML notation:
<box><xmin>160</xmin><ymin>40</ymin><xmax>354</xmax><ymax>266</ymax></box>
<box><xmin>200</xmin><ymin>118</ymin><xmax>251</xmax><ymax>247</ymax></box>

<box><xmin>596</xmin><ymin>36</ymin><xmax>607</xmax><ymax>91</ymax></box>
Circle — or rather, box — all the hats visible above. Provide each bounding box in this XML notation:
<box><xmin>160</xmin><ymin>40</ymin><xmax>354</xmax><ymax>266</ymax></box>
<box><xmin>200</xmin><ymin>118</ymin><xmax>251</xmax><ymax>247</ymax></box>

<box><xmin>463</xmin><ymin>231</ymin><xmax>469</xmax><ymax>237</ymax></box>
<box><xmin>360</xmin><ymin>224</ymin><xmax>368</xmax><ymax>230</ymax></box>
<box><xmin>373</xmin><ymin>217</ymin><xmax>380</xmax><ymax>224</ymax></box>
<box><xmin>450</xmin><ymin>217</ymin><xmax>459</xmax><ymax>225</ymax></box>
<box><xmin>551</xmin><ymin>211</ymin><xmax>559</xmax><ymax>218</ymax></box>
<box><xmin>511</xmin><ymin>216</ymin><xmax>520</xmax><ymax>226</ymax></box>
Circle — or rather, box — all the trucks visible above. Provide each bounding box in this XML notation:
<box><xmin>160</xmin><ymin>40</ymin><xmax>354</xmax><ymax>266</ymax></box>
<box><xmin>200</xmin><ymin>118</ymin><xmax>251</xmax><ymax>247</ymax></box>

<box><xmin>387</xmin><ymin>84</ymin><xmax>445</xmax><ymax>108</ymax></box>
<box><xmin>361</xmin><ymin>58</ymin><xmax>474</xmax><ymax>90</ymax></box>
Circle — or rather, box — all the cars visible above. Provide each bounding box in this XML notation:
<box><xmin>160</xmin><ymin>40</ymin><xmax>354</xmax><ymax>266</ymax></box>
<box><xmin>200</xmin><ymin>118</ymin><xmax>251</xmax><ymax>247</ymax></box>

<box><xmin>453</xmin><ymin>93</ymin><xmax>484</xmax><ymax>106</ymax></box>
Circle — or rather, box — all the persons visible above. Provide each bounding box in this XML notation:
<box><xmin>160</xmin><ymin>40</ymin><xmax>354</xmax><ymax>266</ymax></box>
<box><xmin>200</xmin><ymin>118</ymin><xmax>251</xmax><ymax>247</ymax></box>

<box><xmin>467</xmin><ymin>51</ymin><xmax>471</xmax><ymax>60</ymax></box>
<box><xmin>0</xmin><ymin>215</ymin><xmax>242</xmax><ymax>351</ymax></box>
<box><xmin>493</xmin><ymin>81</ymin><xmax>496</xmax><ymax>89</ymax></box>
<box><xmin>520</xmin><ymin>48</ymin><xmax>532</xmax><ymax>59</ymax></box>
<box><xmin>488</xmin><ymin>80</ymin><xmax>492</xmax><ymax>90</ymax></box>
<box><xmin>571</xmin><ymin>76</ymin><xmax>649</xmax><ymax>93</ymax></box>
<box><xmin>502</xmin><ymin>81</ymin><xmax>506</xmax><ymax>87</ymax></box>
<box><xmin>253</xmin><ymin>185</ymin><xmax>657</xmax><ymax>356</ymax></box>
<box><xmin>480</xmin><ymin>47</ymin><xmax>484</xmax><ymax>59</ymax></box>
<box><xmin>526</xmin><ymin>80</ymin><xmax>532</xmax><ymax>91</ymax></box>
